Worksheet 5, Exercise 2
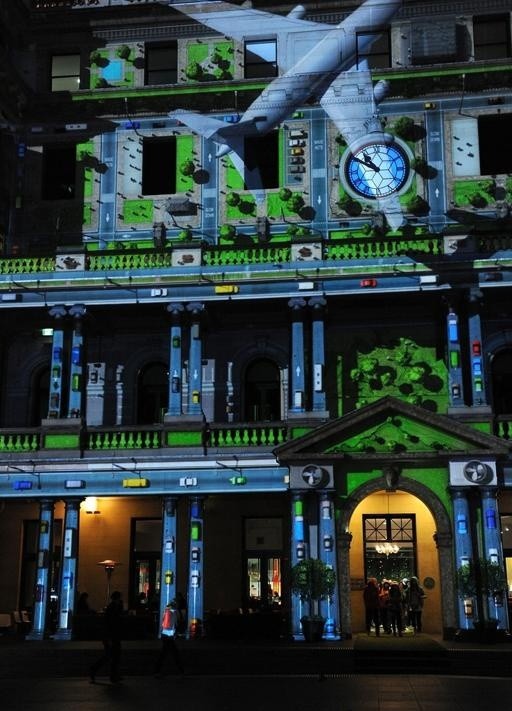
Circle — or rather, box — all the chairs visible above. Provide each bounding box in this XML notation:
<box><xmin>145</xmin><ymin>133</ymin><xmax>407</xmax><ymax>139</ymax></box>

<box><xmin>1</xmin><ymin>611</ymin><xmax>33</xmax><ymax>628</ymax></box>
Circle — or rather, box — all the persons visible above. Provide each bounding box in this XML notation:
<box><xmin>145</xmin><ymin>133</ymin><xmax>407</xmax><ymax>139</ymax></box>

<box><xmin>363</xmin><ymin>576</ymin><xmax>427</xmax><ymax>639</ymax></box>
<box><xmin>88</xmin><ymin>591</ymin><xmax>125</xmax><ymax>684</ymax></box>
<box><xmin>76</xmin><ymin>592</ymin><xmax>97</xmax><ymax>616</ymax></box>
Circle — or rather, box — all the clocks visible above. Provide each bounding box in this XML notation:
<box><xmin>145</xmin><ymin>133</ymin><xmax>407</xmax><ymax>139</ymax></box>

<box><xmin>340</xmin><ymin>133</ymin><xmax>416</xmax><ymax>205</ymax></box>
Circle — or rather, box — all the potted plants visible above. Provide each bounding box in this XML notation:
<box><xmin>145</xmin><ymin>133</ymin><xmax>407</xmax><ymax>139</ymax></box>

<box><xmin>454</xmin><ymin>554</ymin><xmax>510</xmax><ymax>647</ymax></box>
<box><xmin>287</xmin><ymin>555</ymin><xmax>336</xmax><ymax>645</ymax></box>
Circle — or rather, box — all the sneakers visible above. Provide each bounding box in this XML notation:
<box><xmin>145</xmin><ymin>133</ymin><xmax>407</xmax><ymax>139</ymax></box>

<box><xmin>365</xmin><ymin>626</ymin><xmax>421</xmax><ymax>637</ymax></box>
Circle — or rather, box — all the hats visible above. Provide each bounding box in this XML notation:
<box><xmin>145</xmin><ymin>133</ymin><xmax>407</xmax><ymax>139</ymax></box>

<box><xmin>368</xmin><ymin>577</ymin><xmax>410</xmax><ymax>589</ymax></box>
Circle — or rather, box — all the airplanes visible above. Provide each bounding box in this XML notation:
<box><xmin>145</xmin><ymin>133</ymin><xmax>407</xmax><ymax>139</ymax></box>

<box><xmin>159</xmin><ymin>0</ymin><xmax>404</xmax><ymax>233</ymax></box>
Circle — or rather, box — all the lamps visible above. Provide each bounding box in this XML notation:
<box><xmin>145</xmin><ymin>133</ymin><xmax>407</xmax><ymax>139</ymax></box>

<box><xmin>370</xmin><ymin>543</ymin><xmax>399</xmax><ymax>558</ymax></box>
